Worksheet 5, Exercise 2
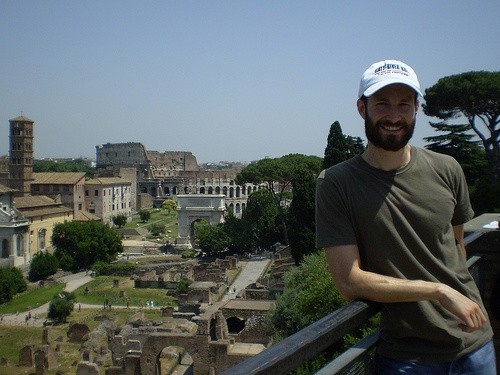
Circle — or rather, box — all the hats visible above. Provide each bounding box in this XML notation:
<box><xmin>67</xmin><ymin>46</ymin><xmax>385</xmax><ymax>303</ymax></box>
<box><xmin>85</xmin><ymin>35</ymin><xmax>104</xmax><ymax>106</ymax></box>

<box><xmin>358</xmin><ymin>60</ymin><xmax>423</xmax><ymax>100</ymax></box>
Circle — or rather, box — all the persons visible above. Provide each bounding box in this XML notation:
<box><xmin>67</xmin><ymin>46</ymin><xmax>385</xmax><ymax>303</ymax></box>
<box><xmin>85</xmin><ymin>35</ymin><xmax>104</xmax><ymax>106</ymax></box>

<box><xmin>315</xmin><ymin>60</ymin><xmax>497</xmax><ymax>375</ymax></box>
